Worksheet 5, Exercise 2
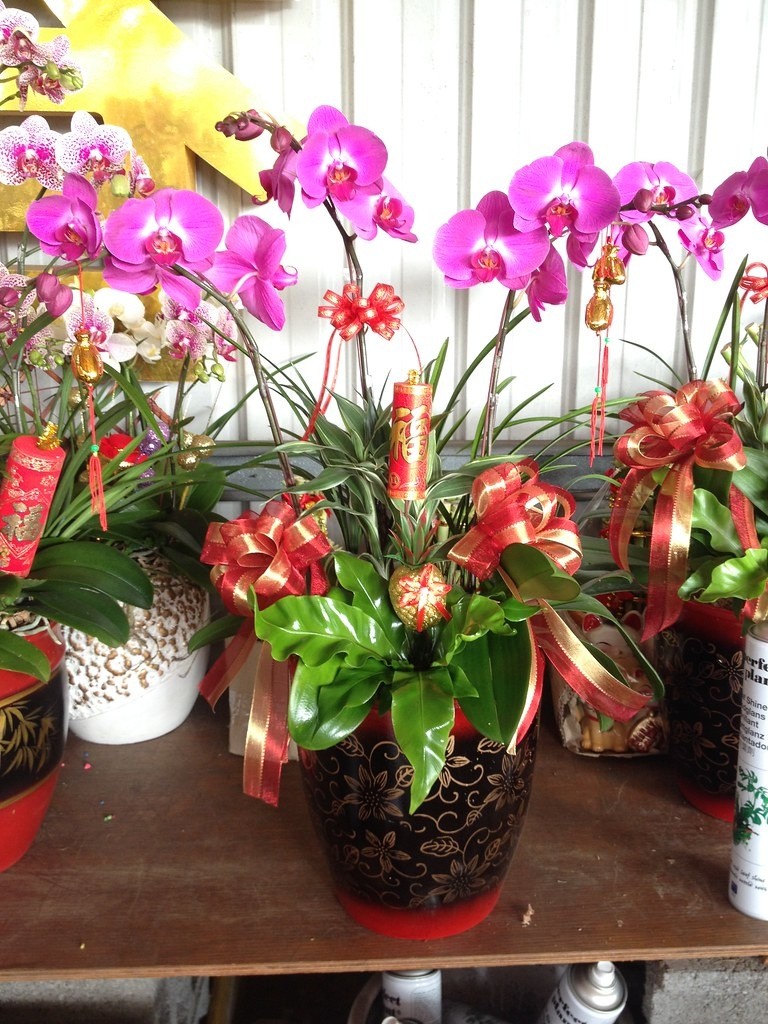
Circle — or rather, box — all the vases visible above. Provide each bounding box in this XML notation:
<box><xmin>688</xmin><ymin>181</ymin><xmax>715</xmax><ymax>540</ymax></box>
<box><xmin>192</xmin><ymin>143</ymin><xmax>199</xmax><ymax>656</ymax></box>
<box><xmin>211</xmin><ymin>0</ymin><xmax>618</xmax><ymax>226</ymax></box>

<box><xmin>0</xmin><ymin>632</ymin><xmax>65</xmax><ymax>872</ymax></box>
<box><xmin>47</xmin><ymin>542</ymin><xmax>212</xmax><ymax>744</ymax></box>
<box><xmin>301</xmin><ymin>658</ymin><xmax>549</xmax><ymax>943</ymax></box>
<box><xmin>671</xmin><ymin>600</ymin><xmax>746</xmax><ymax>822</ymax></box>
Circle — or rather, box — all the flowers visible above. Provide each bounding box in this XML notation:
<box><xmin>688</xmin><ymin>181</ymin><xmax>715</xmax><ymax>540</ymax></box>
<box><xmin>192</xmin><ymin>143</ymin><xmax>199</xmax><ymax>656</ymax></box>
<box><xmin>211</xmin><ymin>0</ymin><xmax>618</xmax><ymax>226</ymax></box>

<box><xmin>0</xmin><ymin>0</ymin><xmax>768</xmax><ymax>821</ymax></box>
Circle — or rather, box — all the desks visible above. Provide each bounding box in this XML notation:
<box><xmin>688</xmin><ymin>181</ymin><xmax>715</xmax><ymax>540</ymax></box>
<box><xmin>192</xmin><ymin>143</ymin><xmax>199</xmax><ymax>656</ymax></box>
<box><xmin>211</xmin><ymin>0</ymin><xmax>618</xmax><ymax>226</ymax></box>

<box><xmin>0</xmin><ymin>706</ymin><xmax>768</xmax><ymax>1024</ymax></box>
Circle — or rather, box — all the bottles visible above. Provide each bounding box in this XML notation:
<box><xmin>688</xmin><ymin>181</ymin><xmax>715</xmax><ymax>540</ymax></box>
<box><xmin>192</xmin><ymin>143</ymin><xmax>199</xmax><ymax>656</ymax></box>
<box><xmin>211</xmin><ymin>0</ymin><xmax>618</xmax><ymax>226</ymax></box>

<box><xmin>367</xmin><ymin>961</ymin><xmax>631</xmax><ymax>1024</ymax></box>
<box><xmin>729</xmin><ymin>580</ymin><xmax>768</xmax><ymax>924</ymax></box>
